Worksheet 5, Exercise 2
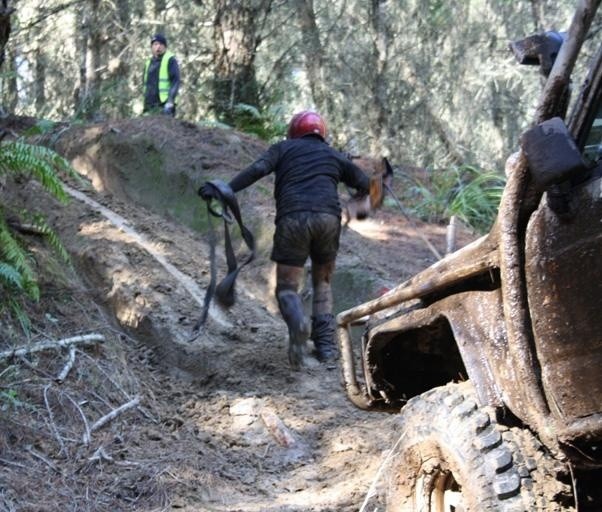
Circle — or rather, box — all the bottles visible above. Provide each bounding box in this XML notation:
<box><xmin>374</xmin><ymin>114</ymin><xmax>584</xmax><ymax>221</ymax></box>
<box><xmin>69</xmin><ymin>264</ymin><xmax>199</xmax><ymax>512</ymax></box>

<box><xmin>150</xmin><ymin>33</ymin><xmax>166</xmax><ymax>45</ymax></box>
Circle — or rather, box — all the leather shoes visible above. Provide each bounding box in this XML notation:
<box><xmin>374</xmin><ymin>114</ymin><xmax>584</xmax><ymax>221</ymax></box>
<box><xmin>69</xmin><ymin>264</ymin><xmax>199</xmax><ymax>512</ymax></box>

<box><xmin>163</xmin><ymin>102</ymin><xmax>175</xmax><ymax>115</ymax></box>
<box><xmin>197</xmin><ymin>183</ymin><xmax>216</xmax><ymax>200</ymax></box>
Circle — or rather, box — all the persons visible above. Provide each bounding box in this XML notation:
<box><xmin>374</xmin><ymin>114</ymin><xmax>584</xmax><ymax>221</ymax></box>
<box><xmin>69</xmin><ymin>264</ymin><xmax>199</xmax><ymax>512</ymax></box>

<box><xmin>140</xmin><ymin>33</ymin><xmax>182</xmax><ymax>117</ymax></box>
<box><xmin>199</xmin><ymin>112</ymin><xmax>371</xmax><ymax>369</ymax></box>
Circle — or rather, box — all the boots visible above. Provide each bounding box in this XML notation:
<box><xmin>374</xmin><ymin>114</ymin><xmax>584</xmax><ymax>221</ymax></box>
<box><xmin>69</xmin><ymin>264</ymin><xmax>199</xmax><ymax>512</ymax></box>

<box><xmin>335</xmin><ymin>2</ymin><xmax>602</xmax><ymax>511</ymax></box>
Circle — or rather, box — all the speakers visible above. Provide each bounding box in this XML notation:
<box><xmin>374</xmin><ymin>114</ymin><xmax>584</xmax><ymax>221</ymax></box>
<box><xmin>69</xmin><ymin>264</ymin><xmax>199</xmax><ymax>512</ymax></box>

<box><xmin>278</xmin><ymin>290</ymin><xmax>311</xmax><ymax>366</ymax></box>
<box><xmin>312</xmin><ymin>313</ymin><xmax>341</xmax><ymax>362</ymax></box>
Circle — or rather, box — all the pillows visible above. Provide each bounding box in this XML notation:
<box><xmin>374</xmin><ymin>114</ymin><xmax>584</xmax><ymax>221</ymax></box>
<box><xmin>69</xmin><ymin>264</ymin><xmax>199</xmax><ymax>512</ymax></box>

<box><xmin>289</xmin><ymin>110</ymin><xmax>326</xmax><ymax>141</ymax></box>
